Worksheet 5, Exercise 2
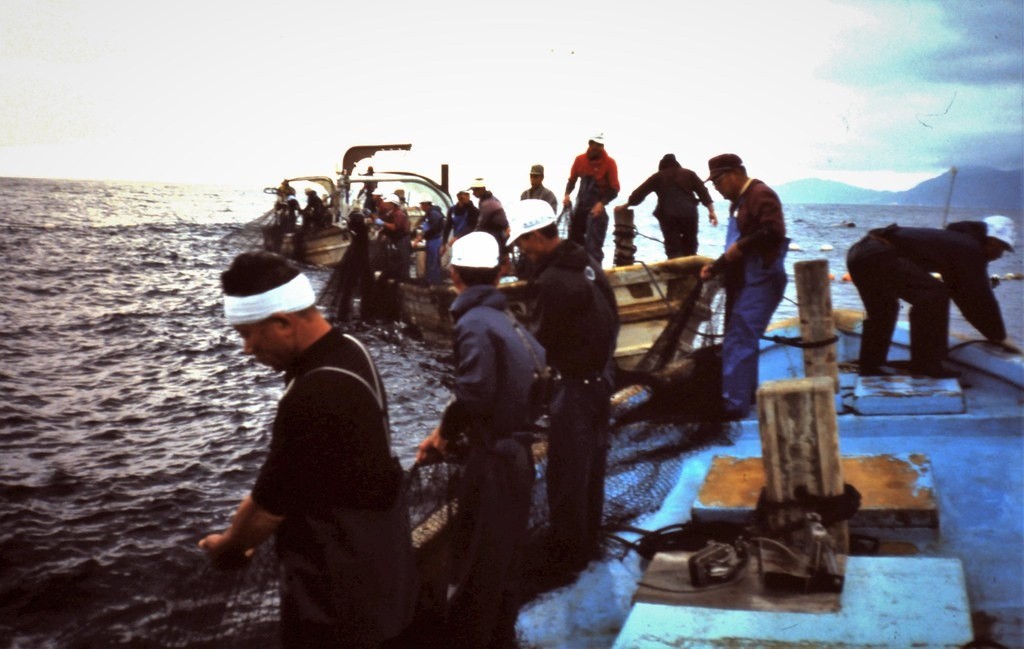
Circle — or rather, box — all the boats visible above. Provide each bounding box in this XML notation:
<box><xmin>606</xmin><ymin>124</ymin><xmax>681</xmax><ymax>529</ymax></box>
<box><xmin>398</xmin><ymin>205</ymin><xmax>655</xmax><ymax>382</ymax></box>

<box><xmin>273</xmin><ymin>143</ymin><xmax>730</xmax><ymax>393</ymax></box>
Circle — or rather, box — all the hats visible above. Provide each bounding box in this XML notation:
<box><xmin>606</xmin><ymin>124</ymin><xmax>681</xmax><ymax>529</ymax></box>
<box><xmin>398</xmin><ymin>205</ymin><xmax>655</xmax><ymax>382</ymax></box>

<box><xmin>450</xmin><ymin>231</ymin><xmax>499</xmax><ymax>268</ymax></box>
<box><xmin>588</xmin><ymin>132</ymin><xmax>604</xmax><ymax>145</ymax></box>
<box><xmin>418</xmin><ymin>196</ymin><xmax>432</xmax><ymax>203</ymax></box>
<box><xmin>704</xmin><ymin>154</ymin><xmax>742</xmax><ymax>183</ymax></box>
<box><xmin>466</xmin><ymin>179</ymin><xmax>486</xmax><ymax>190</ymax></box>
<box><xmin>383</xmin><ymin>194</ymin><xmax>400</xmax><ymax>205</ymax></box>
<box><xmin>984</xmin><ymin>215</ymin><xmax>1017</xmax><ymax>253</ymax></box>
<box><xmin>530</xmin><ymin>165</ymin><xmax>544</xmax><ymax>175</ymax></box>
<box><xmin>506</xmin><ymin>198</ymin><xmax>557</xmax><ymax>247</ymax></box>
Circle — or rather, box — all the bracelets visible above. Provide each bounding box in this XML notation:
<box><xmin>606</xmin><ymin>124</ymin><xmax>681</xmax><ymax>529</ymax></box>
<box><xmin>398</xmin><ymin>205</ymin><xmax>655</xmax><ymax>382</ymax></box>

<box><xmin>713</xmin><ymin>253</ymin><xmax>728</xmax><ymax>271</ymax></box>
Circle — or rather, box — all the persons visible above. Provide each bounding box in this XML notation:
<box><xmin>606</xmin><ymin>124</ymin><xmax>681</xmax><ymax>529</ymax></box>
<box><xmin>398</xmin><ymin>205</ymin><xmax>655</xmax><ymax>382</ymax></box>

<box><xmin>846</xmin><ymin>215</ymin><xmax>1022</xmax><ymax>378</ymax></box>
<box><xmin>700</xmin><ymin>153</ymin><xmax>791</xmax><ymax>421</ymax></box>
<box><xmin>273</xmin><ymin>136</ymin><xmax>719</xmax><ymax>289</ymax></box>
<box><xmin>198</xmin><ymin>252</ymin><xmax>418</xmax><ymax>649</ymax></box>
<box><xmin>416</xmin><ymin>232</ymin><xmax>551</xmax><ymax>649</ymax></box>
<box><xmin>504</xmin><ymin>198</ymin><xmax>619</xmax><ymax>590</ymax></box>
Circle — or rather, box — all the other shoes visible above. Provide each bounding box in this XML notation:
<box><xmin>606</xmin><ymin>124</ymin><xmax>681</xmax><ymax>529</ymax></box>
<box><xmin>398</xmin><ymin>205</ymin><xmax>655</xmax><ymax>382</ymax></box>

<box><xmin>721</xmin><ymin>406</ymin><xmax>745</xmax><ymax>422</ymax></box>
